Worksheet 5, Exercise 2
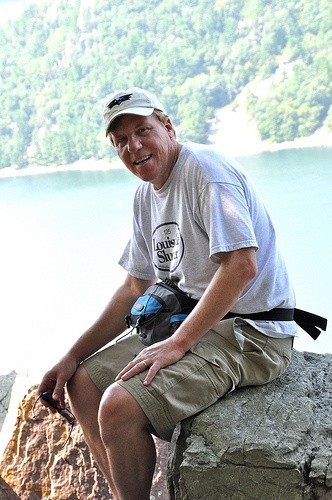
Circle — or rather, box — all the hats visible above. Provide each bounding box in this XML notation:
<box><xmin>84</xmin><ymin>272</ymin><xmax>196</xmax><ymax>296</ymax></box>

<box><xmin>102</xmin><ymin>88</ymin><xmax>164</xmax><ymax>138</ymax></box>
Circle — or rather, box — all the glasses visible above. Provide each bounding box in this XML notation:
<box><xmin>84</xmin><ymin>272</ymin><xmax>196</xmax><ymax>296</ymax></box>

<box><xmin>31</xmin><ymin>391</ymin><xmax>76</xmax><ymax>448</ymax></box>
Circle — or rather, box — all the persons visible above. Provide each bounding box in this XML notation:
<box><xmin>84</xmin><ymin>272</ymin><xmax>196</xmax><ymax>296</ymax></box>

<box><xmin>38</xmin><ymin>84</ymin><xmax>295</xmax><ymax>499</ymax></box>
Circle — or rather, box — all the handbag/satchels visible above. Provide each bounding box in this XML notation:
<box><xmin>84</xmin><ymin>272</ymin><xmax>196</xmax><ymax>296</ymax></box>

<box><xmin>124</xmin><ymin>277</ymin><xmax>191</xmax><ymax>346</ymax></box>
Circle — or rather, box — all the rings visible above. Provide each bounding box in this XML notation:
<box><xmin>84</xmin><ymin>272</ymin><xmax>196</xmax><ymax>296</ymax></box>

<box><xmin>142</xmin><ymin>361</ymin><xmax>148</xmax><ymax>369</ymax></box>
